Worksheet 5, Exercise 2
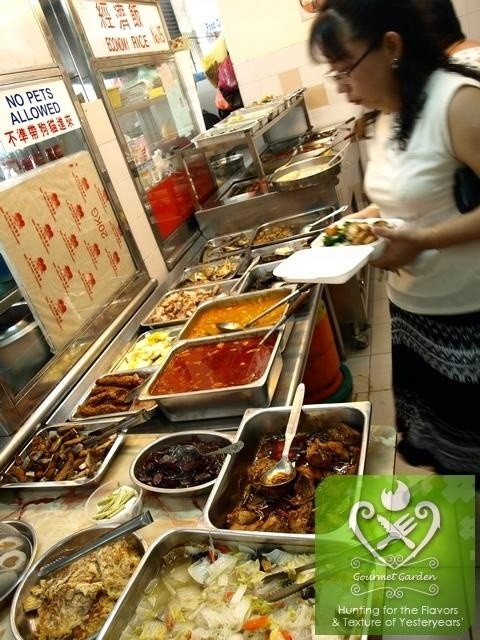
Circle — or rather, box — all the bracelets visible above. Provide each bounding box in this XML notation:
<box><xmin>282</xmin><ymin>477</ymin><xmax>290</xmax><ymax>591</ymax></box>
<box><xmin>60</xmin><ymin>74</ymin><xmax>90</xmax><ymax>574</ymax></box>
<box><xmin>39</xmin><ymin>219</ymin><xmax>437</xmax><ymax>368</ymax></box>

<box><xmin>429</xmin><ymin>226</ymin><xmax>440</xmax><ymax>249</ymax></box>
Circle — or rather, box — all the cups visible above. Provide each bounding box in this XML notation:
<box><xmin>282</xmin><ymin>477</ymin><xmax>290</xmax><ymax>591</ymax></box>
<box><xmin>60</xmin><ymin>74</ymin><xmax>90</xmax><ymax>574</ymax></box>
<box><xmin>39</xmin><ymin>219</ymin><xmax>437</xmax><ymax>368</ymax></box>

<box><xmin>0</xmin><ymin>136</ymin><xmax>65</xmax><ymax>183</ymax></box>
<box><xmin>107</xmin><ymin>87</ymin><xmax>121</xmax><ymax>109</ymax></box>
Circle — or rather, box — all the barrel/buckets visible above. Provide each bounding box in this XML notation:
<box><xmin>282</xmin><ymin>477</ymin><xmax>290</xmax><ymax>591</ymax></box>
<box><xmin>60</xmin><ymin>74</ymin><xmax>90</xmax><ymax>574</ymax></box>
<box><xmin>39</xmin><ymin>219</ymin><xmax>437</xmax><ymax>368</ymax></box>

<box><xmin>326</xmin><ymin>364</ymin><xmax>356</xmax><ymax>401</ymax></box>
<box><xmin>306</xmin><ymin>302</ymin><xmax>341</xmax><ymax>400</ymax></box>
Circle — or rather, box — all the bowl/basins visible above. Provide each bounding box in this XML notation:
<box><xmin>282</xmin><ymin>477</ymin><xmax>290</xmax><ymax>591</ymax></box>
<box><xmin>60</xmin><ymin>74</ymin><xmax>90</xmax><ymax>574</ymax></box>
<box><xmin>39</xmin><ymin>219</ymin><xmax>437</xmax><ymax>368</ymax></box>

<box><xmin>267</xmin><ymin>155</ymin><xmax>344</xmax><ymax>192</ymax></box>
<box><xmin>129</xmin><ymin>430</ymin><xmax>233</xmax><ymax>499</ymax></box>
<box><xmin>86</xmin><ymin>479</ymin><xmax>144</xmax><ymax>527</ymax></box>
<box><xmin>1</xmin><ymin>517</ymin><xmax>38</xmax><ymax>611</ymax></box>
<box><xmin>11</xmin><ymin>522</ymin><xmax>149</xmax><ymax>640</ymax></box>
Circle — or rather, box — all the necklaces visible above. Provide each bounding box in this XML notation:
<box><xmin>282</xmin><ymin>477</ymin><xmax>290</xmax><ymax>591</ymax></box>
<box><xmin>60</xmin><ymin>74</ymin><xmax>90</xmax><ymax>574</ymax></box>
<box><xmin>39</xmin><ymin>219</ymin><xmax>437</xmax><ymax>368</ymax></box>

<box><xmin>447</xmin><ymin>39</ymin><xmax>465</xmax><ymax>56</ymax></box>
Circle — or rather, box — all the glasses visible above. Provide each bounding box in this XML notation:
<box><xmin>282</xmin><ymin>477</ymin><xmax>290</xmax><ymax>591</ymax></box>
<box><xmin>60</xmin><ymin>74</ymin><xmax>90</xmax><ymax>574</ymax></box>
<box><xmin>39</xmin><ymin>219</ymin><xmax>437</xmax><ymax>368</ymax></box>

<box><xmin>321</xmin><ymin>38</ymin><xmax>386</xmax><ymax>82</ymax></box>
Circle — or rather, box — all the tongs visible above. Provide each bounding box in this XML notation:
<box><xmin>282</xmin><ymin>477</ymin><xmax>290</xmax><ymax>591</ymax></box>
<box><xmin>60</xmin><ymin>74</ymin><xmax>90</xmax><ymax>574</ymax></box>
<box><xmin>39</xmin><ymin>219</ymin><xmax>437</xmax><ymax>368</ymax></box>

<box><xmin>79</xmin><ymin>409</ymin><xmax>151</xmax><ymax>451</ymax></box>
<box><xmin>39</xmin><ymin>511</ymin><xmax>153</xmax><ymax>581</ymax></box>
<box><xmin>264</xmin><ymin>531</ymin><xmax>401</xmax><ymax>602</ymax></box>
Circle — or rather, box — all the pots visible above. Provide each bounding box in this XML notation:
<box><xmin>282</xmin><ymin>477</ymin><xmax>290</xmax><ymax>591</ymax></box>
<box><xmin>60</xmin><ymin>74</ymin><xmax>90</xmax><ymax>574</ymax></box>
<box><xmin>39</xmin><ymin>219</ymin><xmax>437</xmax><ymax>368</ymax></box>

<box><xmin>210</xmin><ymin>155</ymin><xmax>247</xmax><ymax>188</ymax></box>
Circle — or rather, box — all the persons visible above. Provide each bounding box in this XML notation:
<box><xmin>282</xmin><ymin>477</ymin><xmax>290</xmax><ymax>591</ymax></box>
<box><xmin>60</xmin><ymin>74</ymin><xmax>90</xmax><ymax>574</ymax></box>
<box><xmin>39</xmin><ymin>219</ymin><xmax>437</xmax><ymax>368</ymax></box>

<box><xmin>308</xmin><ymin>0</ymin><xmax>480</xmax><ymax>493</ymax></box>
<box><xmin>406</xmin><ymin>1</ymin><xmax>480</xmax><ymax>75</ymax></box>
